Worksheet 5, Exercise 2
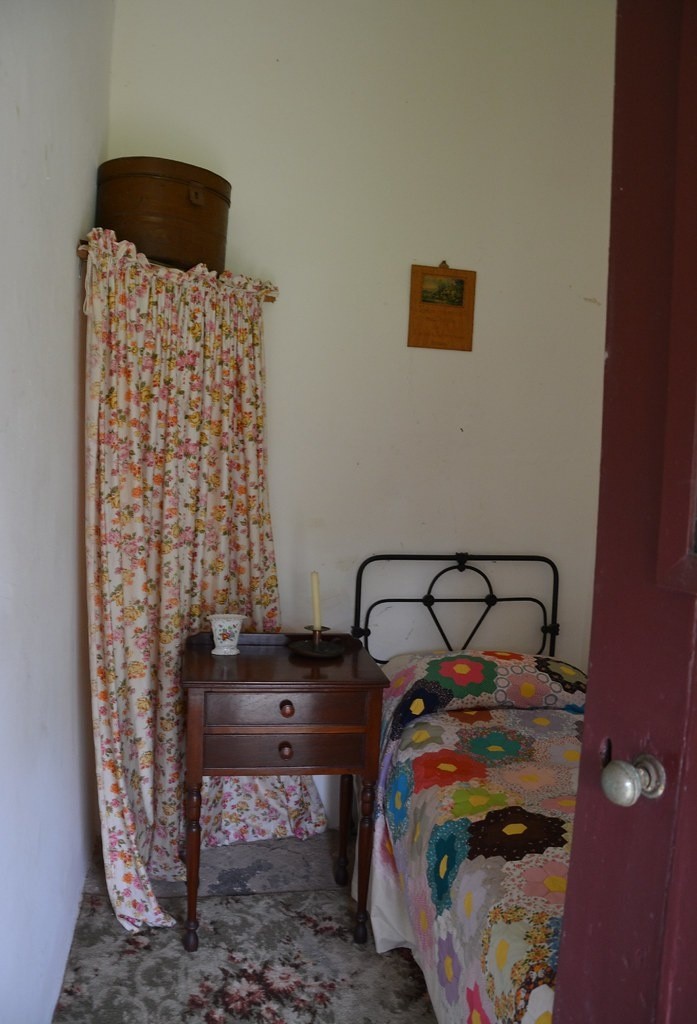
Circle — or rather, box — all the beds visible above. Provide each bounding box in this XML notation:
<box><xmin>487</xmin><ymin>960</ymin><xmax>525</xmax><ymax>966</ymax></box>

<box><xmin>351</xmin><ymin>550</ymin><xmax>589</xmax><ymax>1024</ymax></box>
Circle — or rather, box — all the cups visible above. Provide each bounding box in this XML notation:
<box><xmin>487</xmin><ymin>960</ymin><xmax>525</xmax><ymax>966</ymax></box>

<box><xmin>206</xmin><ymin>614</ymin><xmax>249</xmax><ymax>656</ymax></box>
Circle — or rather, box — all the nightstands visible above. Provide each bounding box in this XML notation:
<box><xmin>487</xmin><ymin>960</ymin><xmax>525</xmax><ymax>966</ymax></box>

<box><xmin>182</xmin><ymin>631</ymin><xmax>391</xmax><ymax>953</ymax></box>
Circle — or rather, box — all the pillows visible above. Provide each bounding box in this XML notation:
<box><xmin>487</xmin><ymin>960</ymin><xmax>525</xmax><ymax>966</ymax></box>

<box><xmin>381</xmin><ymin>648</ymin><xmax>588</xmax><ymax>731</ymax></box>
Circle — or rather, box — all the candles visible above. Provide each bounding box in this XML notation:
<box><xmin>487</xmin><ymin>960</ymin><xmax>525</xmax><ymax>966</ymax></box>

<box><xmin>310</xmin><ymin>571</ymin><xmax>321</xmax><ymax>630</ymax></box>
